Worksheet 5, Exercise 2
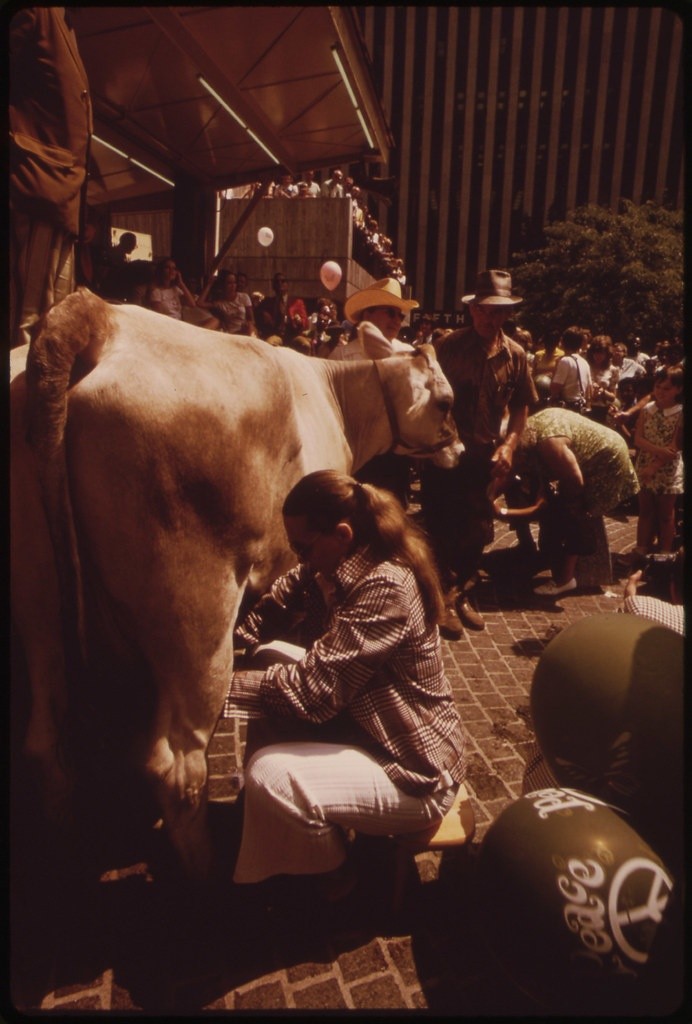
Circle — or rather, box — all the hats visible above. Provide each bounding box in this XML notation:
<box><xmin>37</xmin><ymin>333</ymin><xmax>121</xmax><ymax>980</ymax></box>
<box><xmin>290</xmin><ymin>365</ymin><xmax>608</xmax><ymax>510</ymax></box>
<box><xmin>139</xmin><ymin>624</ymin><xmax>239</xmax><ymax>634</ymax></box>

<box><xmin>461</xmin><ymin>270</ymin><xmax>523</xmax><ymax>305</ymax></box>
<box><xmin>344</xmin><ymin>278</ymin><xmax>419</xmax><ymax>325</ymax></box>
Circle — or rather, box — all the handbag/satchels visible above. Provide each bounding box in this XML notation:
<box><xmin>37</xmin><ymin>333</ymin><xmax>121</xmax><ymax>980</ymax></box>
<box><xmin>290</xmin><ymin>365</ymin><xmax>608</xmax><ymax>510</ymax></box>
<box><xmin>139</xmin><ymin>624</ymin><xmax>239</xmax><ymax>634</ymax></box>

<box><xmin>549</xmin><ymin>397</ymin><xmax>583</xmax><ymax>414</ymax></box>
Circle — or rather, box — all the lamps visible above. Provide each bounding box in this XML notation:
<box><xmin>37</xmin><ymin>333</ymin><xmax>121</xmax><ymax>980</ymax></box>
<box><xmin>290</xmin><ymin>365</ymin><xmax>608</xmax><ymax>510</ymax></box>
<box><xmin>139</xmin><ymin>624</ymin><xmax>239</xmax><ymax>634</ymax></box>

<box><xmin>91</xmin><ymin>133</ymin><xmax>177</xmax><ymax>187</ymax></box>
<box><xmin>195</xmin><ymin>73</ymin><xmax>281</xmax><ymax>164</ymax></box>
<box><xmin>330</xmin><ymin>43</ymin><xmax>376</xmax><ymax>150</ymax></box>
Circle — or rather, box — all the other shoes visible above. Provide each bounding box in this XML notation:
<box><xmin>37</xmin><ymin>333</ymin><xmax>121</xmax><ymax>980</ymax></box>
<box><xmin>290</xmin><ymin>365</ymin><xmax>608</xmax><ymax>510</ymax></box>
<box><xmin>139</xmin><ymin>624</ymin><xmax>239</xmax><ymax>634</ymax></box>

<box><xmin>617</xmin><ymin>550</ymin><xmax>645</xmax><ymax>565</ymax></box>
<box><xmin>437</xmin><ymin>607</ymin><xmax>462</xmax><ymax>635</ymax></box>
<box><xmin>533</xmin><ymin>577</ymin><xmax>577</xmax><ymax>595</ymax></box>
<box><xmin>309</xmin><ymin>870</ymin><xmax>361</xmax><ymax>908</ymax></box>
<box><xmin>459</xmin><ymin>598</ymin><xmax>485</xmax><ymax>630</ymax></box>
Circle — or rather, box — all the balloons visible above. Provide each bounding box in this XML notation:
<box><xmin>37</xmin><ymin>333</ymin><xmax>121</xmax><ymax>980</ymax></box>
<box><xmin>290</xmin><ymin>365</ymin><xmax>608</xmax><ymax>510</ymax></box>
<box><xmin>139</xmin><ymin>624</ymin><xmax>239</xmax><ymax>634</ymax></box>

<box><xmin>320</xmin><ymin>260</ymin><xmax>342</xmax><ymax>291</ymax></box>
<box><xmin>258</xmin><ymin>227</ymin><xmax>274</xmax><ymax>247</ymax></box>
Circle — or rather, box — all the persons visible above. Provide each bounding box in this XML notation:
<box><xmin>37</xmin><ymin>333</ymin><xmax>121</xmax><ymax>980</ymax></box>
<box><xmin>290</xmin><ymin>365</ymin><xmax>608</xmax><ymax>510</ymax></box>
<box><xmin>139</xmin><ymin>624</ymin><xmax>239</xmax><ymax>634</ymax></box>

<box><xmin>80</xmin><ymin>170</ymin><xmax>686</xmax><ymax>640</ymax></box>
<box><xmin>226</xmin><ymin>468</ymin><xmax>468</xmax><ymax>903</ymax></box>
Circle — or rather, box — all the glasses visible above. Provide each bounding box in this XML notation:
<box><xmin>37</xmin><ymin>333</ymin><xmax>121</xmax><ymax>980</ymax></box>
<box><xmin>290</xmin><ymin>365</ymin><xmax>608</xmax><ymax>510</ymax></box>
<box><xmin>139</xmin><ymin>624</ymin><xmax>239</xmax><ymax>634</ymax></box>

<box><xmin>289</xmin><ymin>528</ymin><xmax>335</xmax><ymax>563</ymax></box>
<box><xmin>369</xmin><ymin>308</ymin><xmax>405</xmax><ymax>321</ymax></box>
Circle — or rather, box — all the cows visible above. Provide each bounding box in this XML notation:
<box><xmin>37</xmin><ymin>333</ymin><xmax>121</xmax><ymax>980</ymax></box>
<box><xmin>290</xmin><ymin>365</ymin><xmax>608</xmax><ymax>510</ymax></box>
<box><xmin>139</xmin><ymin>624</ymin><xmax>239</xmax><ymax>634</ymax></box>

<box><xmin>10</xmin><ymin>285</ymin><xmax>464</xmax><ymax>883</ymax></box>
